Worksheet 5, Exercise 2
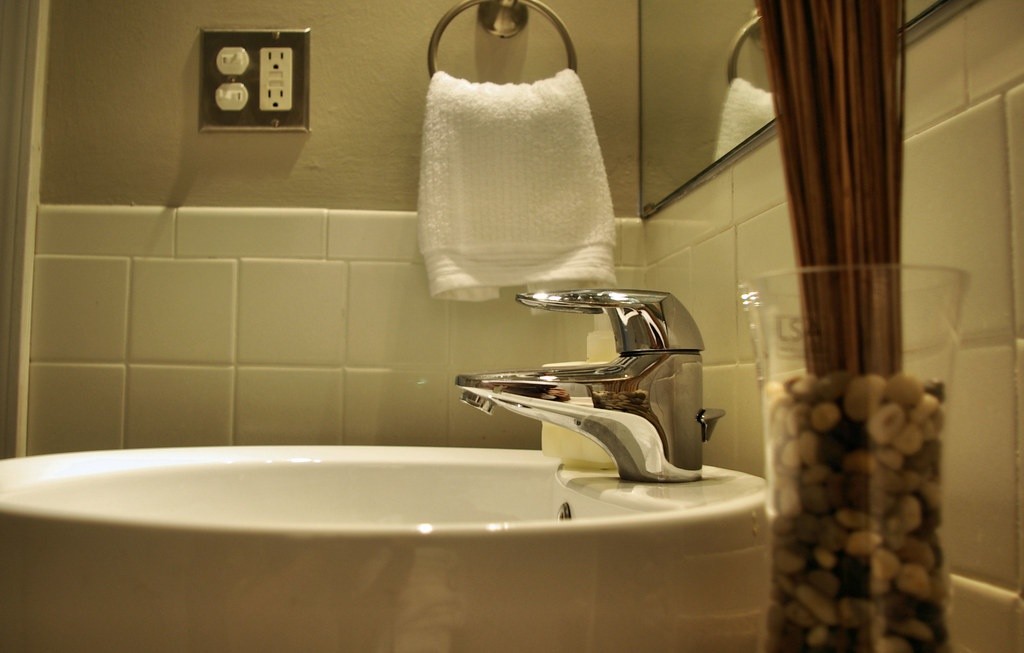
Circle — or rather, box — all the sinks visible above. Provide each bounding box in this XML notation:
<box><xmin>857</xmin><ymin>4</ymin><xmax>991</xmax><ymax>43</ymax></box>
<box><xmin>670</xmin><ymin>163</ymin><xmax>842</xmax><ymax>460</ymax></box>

<box><xmin>0</xmin><ymin>443</ymin><xmax>772</xmax><ymax>653</ymax></box>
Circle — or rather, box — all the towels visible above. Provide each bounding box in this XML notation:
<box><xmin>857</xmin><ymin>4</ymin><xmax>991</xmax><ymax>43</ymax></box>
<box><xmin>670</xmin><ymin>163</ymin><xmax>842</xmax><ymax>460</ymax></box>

<box><xmin>417</xmin><ymin>69</ymin><xmax>620</xmax><ymax>304</ymax></box>
<box><xmin>713</xmin><ymin>77</ymin><xmax>776</xmax><ymax>163</ymax></box>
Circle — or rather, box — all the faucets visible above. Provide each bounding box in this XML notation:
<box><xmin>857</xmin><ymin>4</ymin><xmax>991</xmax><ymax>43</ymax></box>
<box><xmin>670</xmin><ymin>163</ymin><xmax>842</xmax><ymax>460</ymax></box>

<box><xmin>454</xmin><ymin>289</ymin><xmax>727</xmax><ymax>484</ymax></box>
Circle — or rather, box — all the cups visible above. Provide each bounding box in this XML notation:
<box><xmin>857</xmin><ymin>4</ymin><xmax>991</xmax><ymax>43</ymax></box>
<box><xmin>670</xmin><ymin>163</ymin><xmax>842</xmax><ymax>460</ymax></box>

<box><xmin>541</xmin><ymin>361</ymin><xmax>621</xmax><ymax>468</ymax></box>
